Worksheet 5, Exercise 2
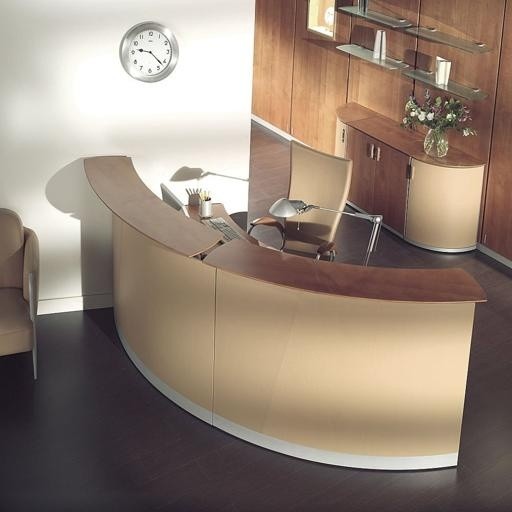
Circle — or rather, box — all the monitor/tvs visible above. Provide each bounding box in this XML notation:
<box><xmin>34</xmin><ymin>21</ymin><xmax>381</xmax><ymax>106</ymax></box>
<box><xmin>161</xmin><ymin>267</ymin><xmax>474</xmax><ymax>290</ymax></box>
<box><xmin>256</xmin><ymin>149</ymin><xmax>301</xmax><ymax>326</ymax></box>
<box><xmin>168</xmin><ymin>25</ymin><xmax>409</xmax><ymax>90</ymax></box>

<box><xmin>160</xmin><ymin>183</ymin><xmax>190</xmax><ymax>218</ymax></box>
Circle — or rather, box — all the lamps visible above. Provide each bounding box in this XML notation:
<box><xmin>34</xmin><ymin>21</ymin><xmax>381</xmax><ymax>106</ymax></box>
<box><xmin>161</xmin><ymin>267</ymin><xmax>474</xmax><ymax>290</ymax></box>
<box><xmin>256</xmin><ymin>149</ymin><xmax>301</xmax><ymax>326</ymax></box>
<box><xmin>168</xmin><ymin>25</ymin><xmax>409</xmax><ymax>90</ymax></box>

<box><xmin>268</xmin><ymin>198</ymin><xmax>382</xmax><ymax>266</ymax></box>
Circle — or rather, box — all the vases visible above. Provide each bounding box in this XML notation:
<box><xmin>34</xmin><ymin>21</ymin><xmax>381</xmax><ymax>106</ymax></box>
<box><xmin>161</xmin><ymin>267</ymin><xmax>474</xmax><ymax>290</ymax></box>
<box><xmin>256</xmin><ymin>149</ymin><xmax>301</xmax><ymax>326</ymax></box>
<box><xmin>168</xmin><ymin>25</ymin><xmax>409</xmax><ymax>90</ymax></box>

<box><xmin>423</xmin><ymin>127</ymin><xmax>448</xmax><ymax>157</ymax></box>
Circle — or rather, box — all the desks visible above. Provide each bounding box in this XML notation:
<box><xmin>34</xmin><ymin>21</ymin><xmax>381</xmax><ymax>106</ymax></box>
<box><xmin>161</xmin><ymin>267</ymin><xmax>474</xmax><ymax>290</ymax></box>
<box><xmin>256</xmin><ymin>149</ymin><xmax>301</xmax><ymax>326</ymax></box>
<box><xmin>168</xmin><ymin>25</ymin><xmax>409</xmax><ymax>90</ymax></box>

<box><xmin>186</xmin><ymin>202</ymin><xmax>272</xmax><ymax>263</ymax></box>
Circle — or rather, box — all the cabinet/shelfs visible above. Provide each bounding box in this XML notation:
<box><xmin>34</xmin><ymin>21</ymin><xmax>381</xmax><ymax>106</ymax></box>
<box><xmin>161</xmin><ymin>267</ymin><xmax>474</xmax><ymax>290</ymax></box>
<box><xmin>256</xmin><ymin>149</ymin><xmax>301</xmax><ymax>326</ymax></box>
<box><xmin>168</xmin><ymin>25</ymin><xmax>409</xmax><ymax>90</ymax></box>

<box><xmin>336</xmin><ymin>102</ymin><xmax>485</xmax><ymax>253</ymax></box>
<box><xmin>336</xmin><ymin>4</ymin><xmax>490</xmax><ymax>104</ymax></box>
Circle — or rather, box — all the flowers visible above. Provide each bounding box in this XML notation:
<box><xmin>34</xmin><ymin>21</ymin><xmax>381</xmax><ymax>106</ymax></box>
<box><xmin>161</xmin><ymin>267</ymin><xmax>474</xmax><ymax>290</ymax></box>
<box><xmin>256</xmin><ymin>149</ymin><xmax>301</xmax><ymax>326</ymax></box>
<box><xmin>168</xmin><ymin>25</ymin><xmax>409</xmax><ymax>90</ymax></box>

<box><xmin>400</xmin><ymin>88</ymin><xmax>478</xmax><ymax>149</ymax></box>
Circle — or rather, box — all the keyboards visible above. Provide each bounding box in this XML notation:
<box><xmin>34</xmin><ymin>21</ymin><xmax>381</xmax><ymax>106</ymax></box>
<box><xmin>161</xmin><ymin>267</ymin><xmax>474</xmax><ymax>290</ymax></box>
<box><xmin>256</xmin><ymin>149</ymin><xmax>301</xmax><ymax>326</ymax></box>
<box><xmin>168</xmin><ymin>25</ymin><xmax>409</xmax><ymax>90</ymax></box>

<box><xmin>201</xmin><ymin>216</ymin><xmax>249</xmax><ymax>244</ymax></box>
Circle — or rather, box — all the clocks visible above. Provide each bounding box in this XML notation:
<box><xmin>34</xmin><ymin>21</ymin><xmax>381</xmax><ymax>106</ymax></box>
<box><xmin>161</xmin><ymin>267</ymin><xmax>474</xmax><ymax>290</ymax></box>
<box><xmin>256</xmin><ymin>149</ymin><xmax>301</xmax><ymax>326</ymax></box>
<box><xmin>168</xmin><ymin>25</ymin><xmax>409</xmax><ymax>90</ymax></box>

<box><xmin>121</xmin><ymin>22</ymin><xmax>179</xmax><ymax>84</ymax></box>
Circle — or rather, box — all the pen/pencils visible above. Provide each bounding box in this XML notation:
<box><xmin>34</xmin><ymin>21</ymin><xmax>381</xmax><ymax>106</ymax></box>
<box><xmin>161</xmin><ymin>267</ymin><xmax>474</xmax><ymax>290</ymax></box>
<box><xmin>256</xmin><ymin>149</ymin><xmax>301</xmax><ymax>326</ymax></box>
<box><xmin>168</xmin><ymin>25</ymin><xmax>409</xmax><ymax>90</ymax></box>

<box><xmin>198</xmin><ymin>190</ymin><xmax>211</xmax><ymax>201</ymax></box>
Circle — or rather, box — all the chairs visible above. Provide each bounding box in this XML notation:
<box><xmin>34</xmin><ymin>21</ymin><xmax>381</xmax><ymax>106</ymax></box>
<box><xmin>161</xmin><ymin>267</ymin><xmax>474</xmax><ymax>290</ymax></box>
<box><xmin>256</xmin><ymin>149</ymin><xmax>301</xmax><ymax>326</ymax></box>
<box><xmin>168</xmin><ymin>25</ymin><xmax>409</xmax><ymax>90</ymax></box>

<box><xmin>248</xmin><ymin>142</ymin><xmax>353</xmax><ymax>262</ymax></box>
<box><xmin>0</xmin><ymin>206</ymin><xmax>41</xmax><ymax>380</ymax></box>
